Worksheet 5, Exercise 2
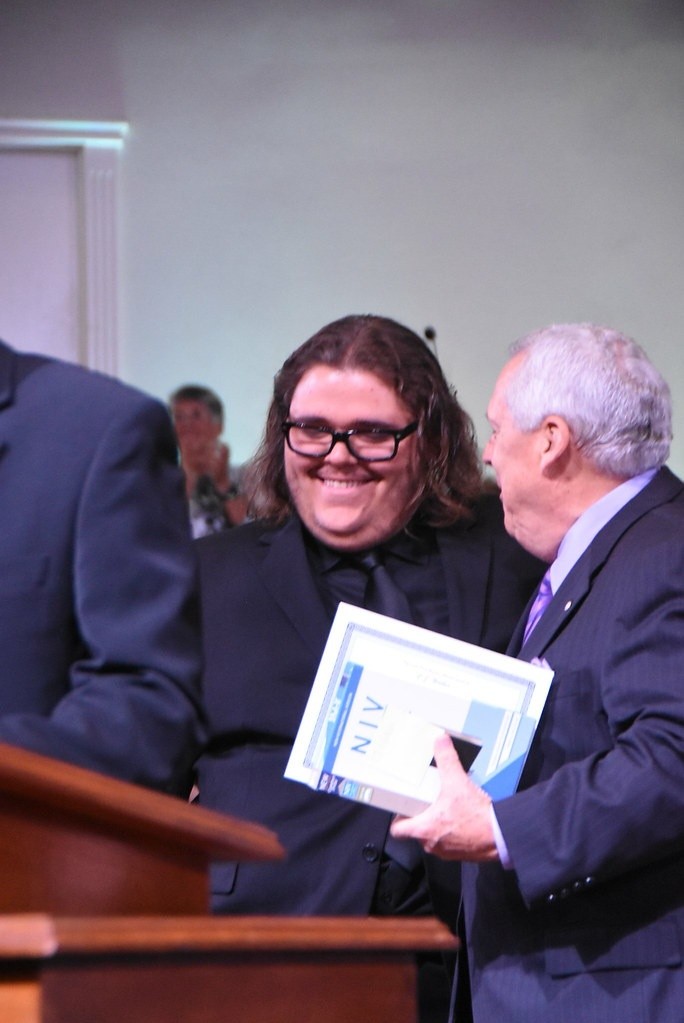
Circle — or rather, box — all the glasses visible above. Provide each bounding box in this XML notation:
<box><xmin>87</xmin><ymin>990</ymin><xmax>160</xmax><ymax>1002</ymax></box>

<box><xmin>282</xmin><ymin>411</ymin><xmax>418</xmax><ymax>463</ymax></box>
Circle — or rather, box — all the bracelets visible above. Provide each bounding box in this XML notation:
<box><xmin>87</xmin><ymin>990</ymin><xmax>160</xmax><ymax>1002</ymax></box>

<box><xmin>222</xmin><ymin>485</ymin><xmax>238</xmax><ymax>500</ymax></box>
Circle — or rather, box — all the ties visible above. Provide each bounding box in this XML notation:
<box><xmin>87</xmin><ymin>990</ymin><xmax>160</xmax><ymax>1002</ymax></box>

<box><xmin>343</xmin><ymin>544</ymin><xmax>414</xmax><ymax>624</ymax></box>
<box><xmin>521</xmin><ymin>567</ymin><xmax>554</xmax><ymax>652</ymax></box>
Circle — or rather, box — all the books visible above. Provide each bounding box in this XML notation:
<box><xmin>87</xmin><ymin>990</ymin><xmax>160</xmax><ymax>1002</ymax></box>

<box><xmin>310</xmin><ymin>664</ymin><xmax>537</xmax><ymax>819</ymax></box>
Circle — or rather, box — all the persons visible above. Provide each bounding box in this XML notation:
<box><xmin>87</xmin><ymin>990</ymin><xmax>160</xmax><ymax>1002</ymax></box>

<box><xmin>189</xmin><ymin>315</ymin><xmax>550</xmax><ymax>915</ymax></box>
<box><xmin>0</xmin><ymin>342</ymin><xmax>200</xmax><ymax>799</ymax></box>
<box><xmin>171</xmin><ymin>384</ymin><xmax>257</xmax><ymax>538</ymax></box>
<box><xmin>391</xmin><ymin>322</ymin><xmax>684</xmax><ymax>1023</ymax></box>
